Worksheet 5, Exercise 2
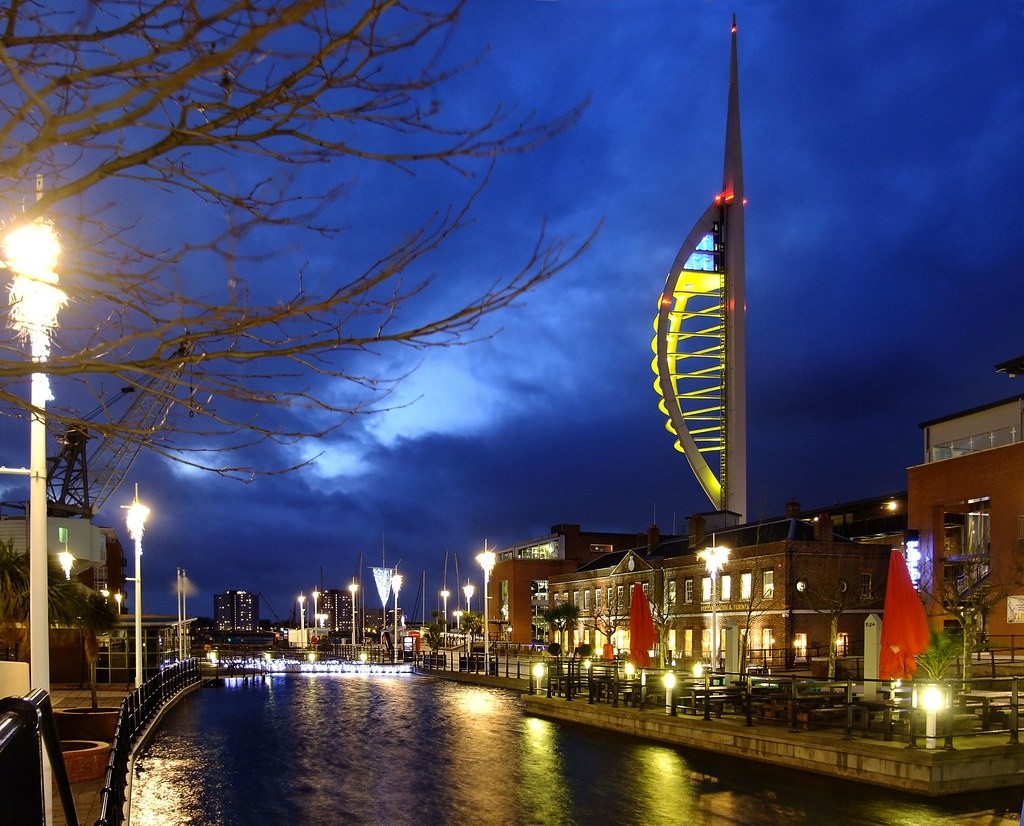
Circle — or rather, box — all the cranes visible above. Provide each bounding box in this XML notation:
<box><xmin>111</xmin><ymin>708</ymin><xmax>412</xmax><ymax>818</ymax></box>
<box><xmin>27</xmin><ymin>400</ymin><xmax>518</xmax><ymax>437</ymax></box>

<box><xmin>259</xmin><ymin>592</ymin><xmax>282</xmax><ymax>625</ymax></box>
<box><xmin>46</xmin><ymin>331</ymin><xmax>197</xmax><ymax>519</ymax></box>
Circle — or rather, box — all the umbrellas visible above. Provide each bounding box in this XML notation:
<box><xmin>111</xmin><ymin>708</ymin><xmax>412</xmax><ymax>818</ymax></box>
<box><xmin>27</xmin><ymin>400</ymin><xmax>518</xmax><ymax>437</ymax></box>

<box><xmin>879</xmin><ymin>551</ymin><xmax>929</xmax><ymax>679</ymax></box>
<box><xmin>629</xmin><ymin>584</ymin><xmax>657</xmax><ymax>668</ymax></box>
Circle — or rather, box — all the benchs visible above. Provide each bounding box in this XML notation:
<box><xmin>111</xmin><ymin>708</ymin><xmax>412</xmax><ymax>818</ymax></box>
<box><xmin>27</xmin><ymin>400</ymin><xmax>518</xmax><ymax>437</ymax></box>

<box><xmin>552</xmin><ymin>683</ymin><xmax>1024</xmax><ymax>735</ymax></box>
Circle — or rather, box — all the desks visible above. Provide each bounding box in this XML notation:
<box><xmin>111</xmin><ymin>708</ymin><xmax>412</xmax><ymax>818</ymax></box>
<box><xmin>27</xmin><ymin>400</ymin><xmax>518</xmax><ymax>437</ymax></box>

<box><xmin>553</xmin><ymin>675</ymin><xmax>1024</xmax><ymax>741</ymax></box>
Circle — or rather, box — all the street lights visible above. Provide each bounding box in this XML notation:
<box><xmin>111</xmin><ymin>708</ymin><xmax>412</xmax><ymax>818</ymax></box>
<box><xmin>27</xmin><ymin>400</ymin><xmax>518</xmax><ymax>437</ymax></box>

<box><xmin>440</xmin><ymin>585</ymin><xmax>448</xmax><ymax>647</ymax></box>
<box><xmin>12</xmin><ymin>174</ymin><xmax>53</xmax><ymax>826</ymax></box>
<box><xmin>702</xmin><ymin>533</ymin><xmax>718</xmax><ymax>671</ymax></box>
<box><xmin>313</xmin><ymin>585</ymin><xmax>317</xmax><ymax>628</ymax></box>
<box><xmin>464</xmin><ymin>578</ymin><xmax>472</xmax><ymax>653</ymax></box>
<box><xmin>482</xmin><ymin>539</ymin><xmax>491</xmax><ymax>672</ymax></box>
<box><xmin>393</xmin><ymin>565</ymin><xmax>399</xmax><ymax>648</ymax></box>
<box><xmin>350</xmin><ymin>577</ymin><xmax>356</xmax><ymax>644</ymax></box>
<box><xmin>116</xmin><ymin>589</ymin><xmax>121</xmax><ymax>615</ymax></box>
<box><xmin>127</xmin><ymin>483</ymin><xmax>144</xmax><ymax>688</ymax></box>
<box><xmin>299</xmin><ymin>591</ymin><xmax>303</xmax><ymax>627</ymax></box>
<box><xmin>453</xmin><ymin>606</ymin><xmax>462</xmax><ymax>629</ymax></box>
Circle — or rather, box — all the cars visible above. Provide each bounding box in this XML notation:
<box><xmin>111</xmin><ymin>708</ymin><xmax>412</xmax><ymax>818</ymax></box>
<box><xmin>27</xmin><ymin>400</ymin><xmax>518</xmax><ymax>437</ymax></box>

<box><xmin>351</xmin><ymin>660</ymin><xmax>364</xmax><ymax>665</ymax></box>
<box><xmin>223</xmin><ymin>633</ymin><xmax>240</xmax><ymax>643</ymax></box>
